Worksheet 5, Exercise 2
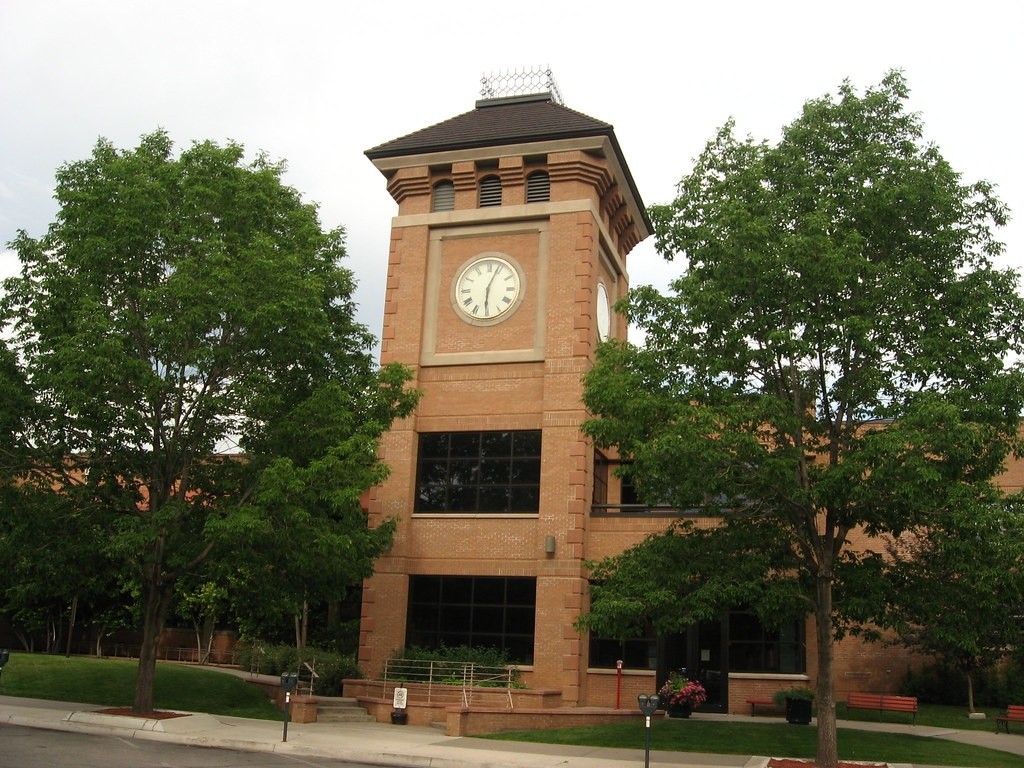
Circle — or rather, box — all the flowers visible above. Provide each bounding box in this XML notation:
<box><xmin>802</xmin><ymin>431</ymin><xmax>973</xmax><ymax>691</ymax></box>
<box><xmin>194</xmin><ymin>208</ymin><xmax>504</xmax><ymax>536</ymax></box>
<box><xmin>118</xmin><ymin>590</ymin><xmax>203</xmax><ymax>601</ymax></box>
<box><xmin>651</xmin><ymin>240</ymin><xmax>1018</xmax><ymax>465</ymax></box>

<box><xmin>658</xmin><ymin>670</ymin><xmax>707</xmax><ymax>710</ymax></box>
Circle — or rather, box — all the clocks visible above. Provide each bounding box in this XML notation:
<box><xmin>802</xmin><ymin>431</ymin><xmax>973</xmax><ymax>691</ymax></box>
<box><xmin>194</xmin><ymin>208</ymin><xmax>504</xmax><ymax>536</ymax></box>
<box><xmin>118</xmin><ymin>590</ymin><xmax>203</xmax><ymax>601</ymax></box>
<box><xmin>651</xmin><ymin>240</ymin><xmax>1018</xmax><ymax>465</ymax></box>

<box><xmin>449</xmin><ymin>251</ymin><xmax>526</xmax><ymax>327</ymax></box>
<box><xmin>596</xmin><ymin>282</ymin><xmax>610</xmax><ymax>343</ymax></box>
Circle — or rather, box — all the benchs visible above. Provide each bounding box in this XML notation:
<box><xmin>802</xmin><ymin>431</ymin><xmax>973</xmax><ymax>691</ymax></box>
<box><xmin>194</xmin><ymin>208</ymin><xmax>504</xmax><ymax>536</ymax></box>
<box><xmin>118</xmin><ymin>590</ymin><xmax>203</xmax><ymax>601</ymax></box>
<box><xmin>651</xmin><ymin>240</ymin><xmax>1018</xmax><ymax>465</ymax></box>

<box><xmin>846</xmin><ymin>694</ymin><xmax>918</xmax><ymax>726</ymax></box>
<box><xmin>995</xmin><ymin>705</ymin><xmax>1024</xmax><ymax>734</ymax></box>
<box><xmin>745</xmin><ymin>696</ymin><xmax>774</xmax><ymax>717</ymax></box>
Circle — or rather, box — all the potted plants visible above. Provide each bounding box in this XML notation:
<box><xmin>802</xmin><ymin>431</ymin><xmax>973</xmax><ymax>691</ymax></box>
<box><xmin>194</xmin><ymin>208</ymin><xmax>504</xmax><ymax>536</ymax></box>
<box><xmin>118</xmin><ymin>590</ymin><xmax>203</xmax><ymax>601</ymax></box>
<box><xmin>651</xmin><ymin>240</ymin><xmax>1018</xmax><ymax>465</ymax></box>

<box><xmin>772</xmin><ymin>685</ymin><xmax>815</xmax><ymax>725</ymax></box>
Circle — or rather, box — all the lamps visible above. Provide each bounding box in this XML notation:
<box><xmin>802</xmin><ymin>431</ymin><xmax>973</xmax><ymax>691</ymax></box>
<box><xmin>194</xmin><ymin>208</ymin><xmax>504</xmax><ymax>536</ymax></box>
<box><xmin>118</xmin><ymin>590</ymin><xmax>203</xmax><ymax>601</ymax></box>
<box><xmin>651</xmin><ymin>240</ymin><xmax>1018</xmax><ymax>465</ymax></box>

<box><xmin>545</xmin><ymin>536</ymin><xmax>555</xmax><ymax>558</ymax></box>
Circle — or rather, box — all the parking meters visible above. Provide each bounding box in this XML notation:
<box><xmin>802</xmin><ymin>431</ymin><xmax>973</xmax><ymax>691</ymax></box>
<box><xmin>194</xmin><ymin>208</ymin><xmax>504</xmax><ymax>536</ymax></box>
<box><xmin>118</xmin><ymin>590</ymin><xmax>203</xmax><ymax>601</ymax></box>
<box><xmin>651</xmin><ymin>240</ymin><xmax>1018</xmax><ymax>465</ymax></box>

<box><xmin>637</xmin><ymin>693</ymin><xmax>661</xmax><ymax>768</ymax></box>
<box><xmin>280</xmin><ymin>672</ymin><xmax>298</xmax><ymax>742</ymax></box>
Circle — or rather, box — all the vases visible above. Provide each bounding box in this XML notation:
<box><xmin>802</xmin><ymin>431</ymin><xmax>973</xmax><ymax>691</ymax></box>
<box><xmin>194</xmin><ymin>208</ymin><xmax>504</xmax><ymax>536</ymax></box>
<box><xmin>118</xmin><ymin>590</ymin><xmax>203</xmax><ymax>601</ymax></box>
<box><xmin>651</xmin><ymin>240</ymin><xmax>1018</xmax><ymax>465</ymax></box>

<box><xmin>667</xmin><ymin>706</ymin><xmax>692</xmax><ymax>719</ymax></box>
